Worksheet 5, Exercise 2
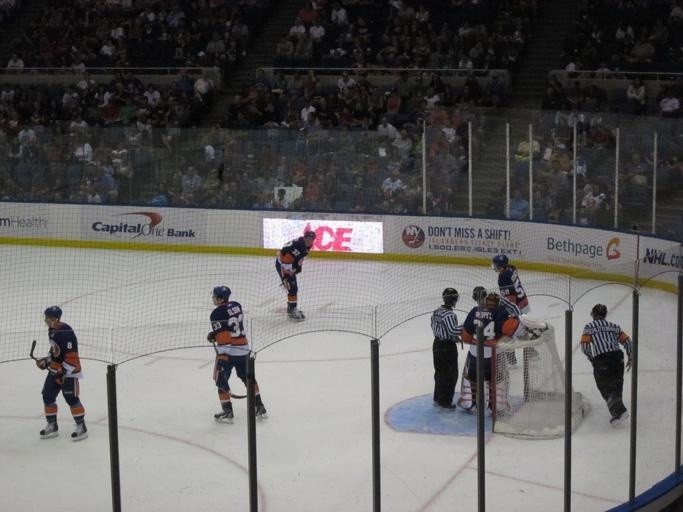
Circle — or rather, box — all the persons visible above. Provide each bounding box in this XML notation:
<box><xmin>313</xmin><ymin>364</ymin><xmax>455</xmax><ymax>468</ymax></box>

<box><xmin>577</xmin><ymin>301</ymin><xmax>633</xmax><ymax>421</ymax></box>
<box><xmin>430</xmin><ymin>288</ymin><xmax>468</xmax><ymax>406</ymax></box>
<box><xmin>0</xmin><ymin>3</ymin><xmax>683</xmax><ymax>246</ymax></box>
<box><xmin>471</xmin><ymin>287</ymin><xmax>524</xmax><ymax>401</ymax></box>
<box><xmin>491</xmin><ymin>253</ymin><xmax>542</xmax><ymax>366</ymax></box>
<box><xmin>34</xmin><ymin>304</ymin><xmax>91</xmax><ymax>439</ymax></box>
<box><xmin>460</xmin><ymin>293</ymin><xmax>517</xmax><ymax>410</ymax></box>
<box><xmin>208</xmin><ymin>285</ymin><xmax>269</xmax><ymax>418</ymax></box>
<box><xmin>273</xmin><ymin>231</ymin><xmax>318</xmax><ymax>319</ymax></box>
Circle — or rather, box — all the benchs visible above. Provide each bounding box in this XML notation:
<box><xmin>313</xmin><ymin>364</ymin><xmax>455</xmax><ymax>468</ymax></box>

<box><xmin>0</xmin><ymin>91</ymin><xmax>681</xmax><ymax>240</ymax></box>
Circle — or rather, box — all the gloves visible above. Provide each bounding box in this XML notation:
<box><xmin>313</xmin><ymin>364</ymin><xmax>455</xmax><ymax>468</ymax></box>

<box><xmin>36</xmin><ymin>358</ymin><xmax>48</xmax><ymax>369</ymax></box>
<box><xmin>208</xmin><ymin>332</ymin><xmax>216</xmax><ymax>342</ymax></box>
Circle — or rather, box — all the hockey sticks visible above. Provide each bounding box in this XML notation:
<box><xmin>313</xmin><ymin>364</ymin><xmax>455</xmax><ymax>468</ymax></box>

<box><xmin>30</xmin><ymin>341</ymin><xmax>52</xmax><ymax>371</ymax></box>
<box><xmin>279</xmin><ymin>270</ymin><xmax>295</xmax><ymax>294</ymax></box>
<box><xmin>211</xmin><ymin>339</ymin><xmax>247</xmax><ymax>399</ymax></box>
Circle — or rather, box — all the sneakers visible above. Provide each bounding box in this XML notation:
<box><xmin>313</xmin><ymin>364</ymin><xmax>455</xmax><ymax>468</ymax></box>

<box><xmin>434</xmin><ymin>399</ymin><xmax>455</xmax><ymax>408</ymax></box>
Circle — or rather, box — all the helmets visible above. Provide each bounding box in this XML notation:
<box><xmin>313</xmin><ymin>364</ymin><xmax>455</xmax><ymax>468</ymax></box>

<box><xmin>592</xmin><ymin>305</ymin><xmax>607</xmax><ymax>318</ymax></box>
<box><xmin>304</xmin><ymin>231</ymin><xmax>315</xmax><ymax>238</ymax></box>
<box><xmin>472</xmin><ymin>287</ymin><xmax>499</xmax><ymax>308</ymax></box>
<box><xmin>213</xmin><ymin>287</ymin><xmax>231</xmax><ymax>298</ymax></box>
<box><xmin>442</xmin><ymin>288</ymin><xmax>458</xmax><ymax>306</ymax></box>
<box><xmin>45</xmin><ymin>306</ymin><xmax>62</xmax><ymax>318</ymax></box>
<box><xmin>492</xmin><ymin>255</ymin><xmax>508</xmax><ymax>272</ymax></box>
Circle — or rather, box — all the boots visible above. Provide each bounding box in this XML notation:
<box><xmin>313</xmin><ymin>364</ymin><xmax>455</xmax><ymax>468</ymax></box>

<box><xmin>40</xmin><ymin>415</ymin><xmax>58</xmax><ymax>436</ymax></box>
<box><xmin>287</xmin><ymin>302</ymin><xmax>304</xmax><ymax>319</ymax></box>
<box><xmin>607</xmin><ymin>399</ymin><xmax>626</xmax><ymax>423</ymax></box>
<box><xmin>215</xmin><ymin>402</ymin><xmax>233</xmax><ymax>419</ymax></box>
<box><xmin>254</xmin><ymin>394</ymin><xmax>266</xmax><ymax>416</ymax></box>
<box><xmin>71</xmin><ymin>415</ymin><xmax>86</xmax><ymax>437</ymax></box>
